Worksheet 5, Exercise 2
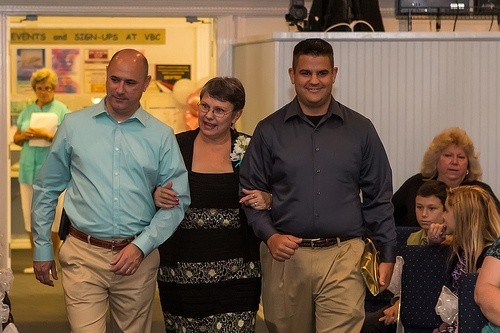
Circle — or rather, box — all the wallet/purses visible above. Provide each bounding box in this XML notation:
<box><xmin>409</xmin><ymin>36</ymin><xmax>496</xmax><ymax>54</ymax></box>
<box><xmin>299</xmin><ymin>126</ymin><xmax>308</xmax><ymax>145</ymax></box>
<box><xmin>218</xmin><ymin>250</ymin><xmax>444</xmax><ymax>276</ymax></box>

<box><xmin>360</xmin><ymin>238</ymin><xmax>380</xmax><ymax>296</ymax></box>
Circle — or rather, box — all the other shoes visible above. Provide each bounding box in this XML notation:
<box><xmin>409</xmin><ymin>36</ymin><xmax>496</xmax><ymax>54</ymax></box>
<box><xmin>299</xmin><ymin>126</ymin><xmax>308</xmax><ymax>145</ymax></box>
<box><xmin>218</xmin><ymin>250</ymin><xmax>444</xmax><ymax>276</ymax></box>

<box><xmin>23</xmin><ymin>267</ymin><xmax>58</xmax><ymax>274</ymax></box>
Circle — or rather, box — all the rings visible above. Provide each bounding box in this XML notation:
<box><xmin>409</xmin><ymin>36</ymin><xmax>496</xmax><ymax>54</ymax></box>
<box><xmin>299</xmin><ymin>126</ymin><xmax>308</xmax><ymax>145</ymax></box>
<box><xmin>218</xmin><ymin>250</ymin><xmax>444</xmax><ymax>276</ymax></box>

<box><xmin>253</xmin><ymin>194</ymin><xmax>255</xmax><ymax>198</ymax></box>
<box><xmin>254</xmin><ymin>202</ymin><xmax>257</xmax><ymax>206</ymax></box>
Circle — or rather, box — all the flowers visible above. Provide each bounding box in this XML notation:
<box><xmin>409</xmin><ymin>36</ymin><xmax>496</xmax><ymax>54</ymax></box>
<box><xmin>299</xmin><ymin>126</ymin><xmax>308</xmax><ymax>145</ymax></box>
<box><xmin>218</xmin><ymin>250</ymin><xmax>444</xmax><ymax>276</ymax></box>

<box><xmin>230</xmin><ymin>135</ymin><xmax>252</xmax><ymax>168</ymax></box>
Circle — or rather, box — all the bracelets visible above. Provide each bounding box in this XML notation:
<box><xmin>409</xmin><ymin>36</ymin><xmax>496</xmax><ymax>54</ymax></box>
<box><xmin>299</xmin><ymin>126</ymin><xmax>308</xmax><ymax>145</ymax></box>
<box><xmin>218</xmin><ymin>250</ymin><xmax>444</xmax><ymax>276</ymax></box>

<box><xmin>268</xmin><ymin>194</ymin><xmax>273</xmax><ymax>210</ymax></box>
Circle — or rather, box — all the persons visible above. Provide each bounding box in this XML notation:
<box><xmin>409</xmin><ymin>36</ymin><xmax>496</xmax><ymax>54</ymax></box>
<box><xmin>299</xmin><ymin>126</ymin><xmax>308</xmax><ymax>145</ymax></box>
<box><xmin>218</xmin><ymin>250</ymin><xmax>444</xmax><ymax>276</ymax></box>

<box><xmin>428</xmin><ymin>185</ymin><xmax>500</xmax><ymax>333</ymax></box>
<box><xmin>474</xmin><ymin>236</ymin><xmax>500</xmax><ymax>333</ymax></box>
<box><xmin>153</xmin><ymin>77</ymin><xmax>272</xmax><ymax>333</ymax></box>
<box><xmin>392</xmin><ymin>127</ymin><xmax>500</xmax><ymax>227</ymax></box>
<box><xmin>31</xmin><ymin>49</ymin><xmax>191</xmax><ymax>332</ymax></box>
<box><xmin>379</xmin><ymin>180</ymin><xmax>451</xmax><ymax>325</ymax></box>
<box><xmin>13</xmin><ymin>68</ymin><xmax>71</xmax><ymax>274</ymax></box>
<box><xmin>239</xmin><ymin>39</ymin><xmax>397</xmax><ymax>333</ymax></box>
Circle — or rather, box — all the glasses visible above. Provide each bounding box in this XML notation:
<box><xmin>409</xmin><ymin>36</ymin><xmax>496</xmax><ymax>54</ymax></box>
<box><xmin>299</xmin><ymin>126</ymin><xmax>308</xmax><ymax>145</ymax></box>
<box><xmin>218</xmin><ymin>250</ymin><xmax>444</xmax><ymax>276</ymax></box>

<box><xmin>197</xmin><ymin>104</ymin><xmax>232</xmax><ymax>118</ymax></box>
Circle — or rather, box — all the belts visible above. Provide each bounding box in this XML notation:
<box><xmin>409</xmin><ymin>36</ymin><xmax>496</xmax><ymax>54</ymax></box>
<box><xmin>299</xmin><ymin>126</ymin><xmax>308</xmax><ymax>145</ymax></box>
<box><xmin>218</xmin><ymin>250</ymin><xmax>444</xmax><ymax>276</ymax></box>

<box><xmin>69</xmin><ymin>225</ymin><xmax>134</xmax><ymax>250</ymax></box>
<box><xmin>298</xmin><ymin>235</ymin><xmax>361</xmax><ymax>248</ymax></box>
